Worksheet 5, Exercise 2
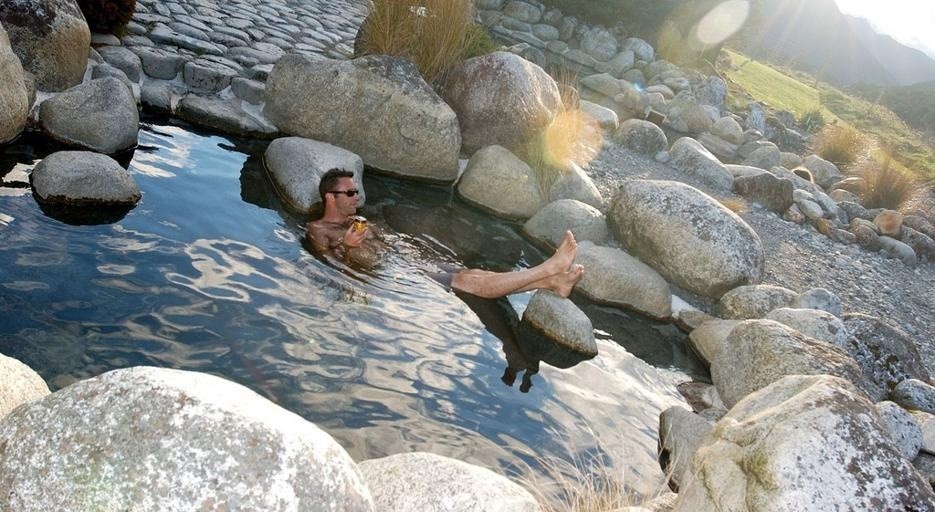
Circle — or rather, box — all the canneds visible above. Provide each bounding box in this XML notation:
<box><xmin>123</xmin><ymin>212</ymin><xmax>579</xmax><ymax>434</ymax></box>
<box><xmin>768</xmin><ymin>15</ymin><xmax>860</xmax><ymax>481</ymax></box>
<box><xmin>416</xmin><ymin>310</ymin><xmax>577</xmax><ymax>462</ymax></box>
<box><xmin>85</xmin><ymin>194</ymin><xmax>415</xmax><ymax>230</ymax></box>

<box><xmin>353</xmin><ymin>216</ymin><xmax>367</xmax><ymax>231</ymax></box>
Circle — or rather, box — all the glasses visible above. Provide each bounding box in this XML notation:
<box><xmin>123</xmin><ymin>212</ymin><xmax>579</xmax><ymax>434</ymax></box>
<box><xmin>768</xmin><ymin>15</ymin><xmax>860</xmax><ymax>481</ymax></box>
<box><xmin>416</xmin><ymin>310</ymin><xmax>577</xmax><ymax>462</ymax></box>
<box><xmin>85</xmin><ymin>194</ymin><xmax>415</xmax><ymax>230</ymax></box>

<box><xmin>325</xmin><ymin>191</ymin><xmax>358</xmax><ymax>197</ymax></box>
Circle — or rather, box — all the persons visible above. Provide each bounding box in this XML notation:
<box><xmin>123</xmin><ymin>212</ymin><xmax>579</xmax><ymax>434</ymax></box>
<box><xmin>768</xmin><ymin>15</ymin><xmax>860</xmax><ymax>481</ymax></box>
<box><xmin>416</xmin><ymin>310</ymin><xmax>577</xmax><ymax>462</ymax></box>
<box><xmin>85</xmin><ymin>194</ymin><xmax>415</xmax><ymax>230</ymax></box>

<box><xmin>305</xmin><ymin>166</ymin><xmax>586</xmax><ymax>299</ymax></box>
